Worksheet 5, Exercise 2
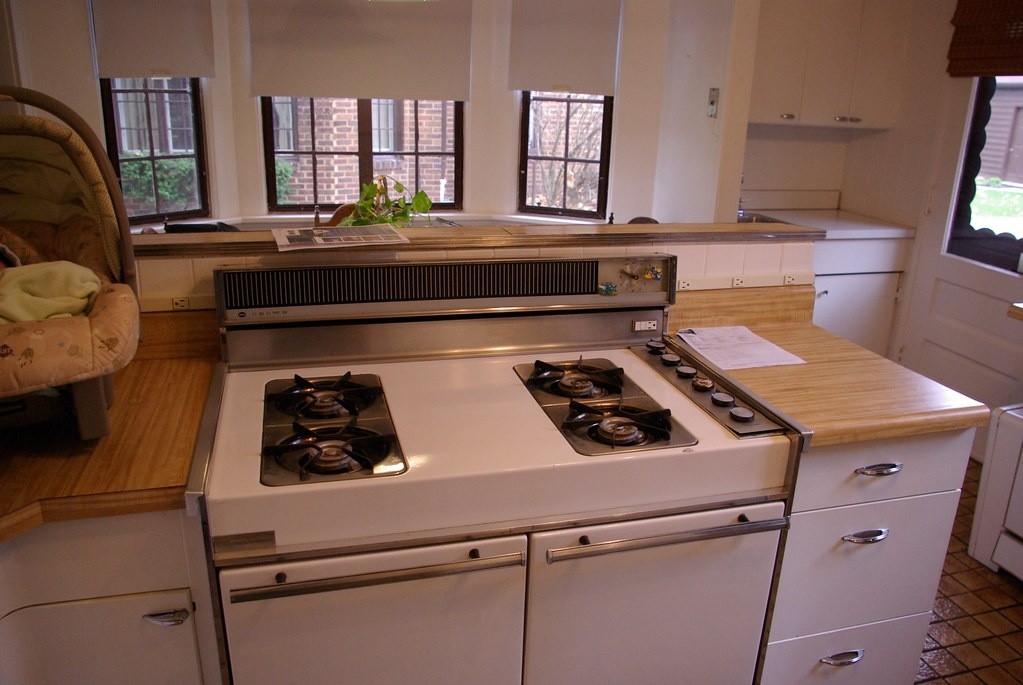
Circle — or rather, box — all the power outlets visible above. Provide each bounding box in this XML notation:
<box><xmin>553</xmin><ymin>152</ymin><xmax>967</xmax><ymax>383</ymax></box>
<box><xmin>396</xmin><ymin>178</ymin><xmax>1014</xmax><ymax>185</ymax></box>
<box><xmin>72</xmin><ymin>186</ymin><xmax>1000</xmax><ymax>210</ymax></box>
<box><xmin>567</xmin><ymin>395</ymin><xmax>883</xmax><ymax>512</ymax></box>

<box><xmin>677</xmin><ymin>280</ymin><xmax>693</xmax><ymax>290</ymax></box>
<box><xmin>172</xmin><ymin>298</ymin><xmax>189</xmax><ymax>311</ymax></box>
<box><xmin>732</xmin><ymin>277</ymin><xmax>744</xmax><ymax>288</ymax></box>
<box><xmin>784</xmin><ymin>275</ymin><xmax>796</xmax><ymax>284</ymax></box>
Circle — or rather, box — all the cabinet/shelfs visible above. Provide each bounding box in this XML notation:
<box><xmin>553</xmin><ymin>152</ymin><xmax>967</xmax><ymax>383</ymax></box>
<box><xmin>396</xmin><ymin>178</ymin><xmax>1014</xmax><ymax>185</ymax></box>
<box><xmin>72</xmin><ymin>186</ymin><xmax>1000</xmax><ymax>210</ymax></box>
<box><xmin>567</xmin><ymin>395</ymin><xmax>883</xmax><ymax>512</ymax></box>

<box><xmin>664</xmin><ymin>328</ymin><xmax>990</xmax><ymax>685</ymax></box>
<box><xmin>814</xmin><ymin>239</ymin><xmax>913</xmax><ymax>358</ymax></box>
<box><xmin>0</xmin><ymin>356</ymin><xmax>225</xmax><ymax>685</ymax></box>
<box><xmin>748</xmin><ymin>1</ymin><xmax>917</xmax><ymax>130</ymax></box>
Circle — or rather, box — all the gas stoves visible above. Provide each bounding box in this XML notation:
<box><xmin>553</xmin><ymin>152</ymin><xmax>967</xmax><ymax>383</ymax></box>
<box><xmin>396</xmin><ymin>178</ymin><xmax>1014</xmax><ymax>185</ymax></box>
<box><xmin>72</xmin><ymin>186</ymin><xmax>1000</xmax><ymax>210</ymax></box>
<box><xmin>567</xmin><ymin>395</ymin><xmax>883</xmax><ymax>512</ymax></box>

<box><xmin>200</xmin><ymin>252</ymin><xmax>811</xmax><ymax>568</ymax></box>
